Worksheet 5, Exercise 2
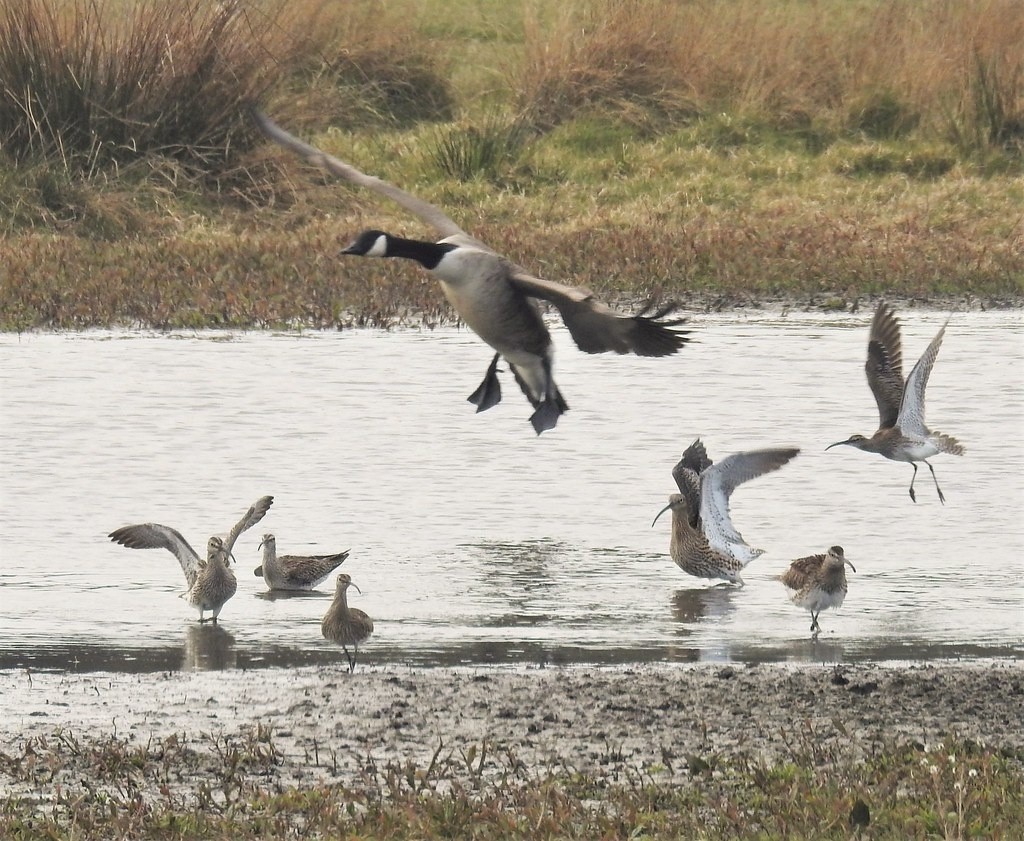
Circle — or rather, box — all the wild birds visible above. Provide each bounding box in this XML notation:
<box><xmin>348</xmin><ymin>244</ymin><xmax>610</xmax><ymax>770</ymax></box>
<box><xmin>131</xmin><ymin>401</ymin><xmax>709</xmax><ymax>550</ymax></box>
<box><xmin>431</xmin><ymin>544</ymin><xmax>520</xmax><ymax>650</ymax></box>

<box><xmin>108</xmin><ymin>494</ymin><xmax>375</xmax><ymax>678</ymax></box>
<box><xmin>779</xmin><ymin>546</ymin><xmax>856</xmax><ymax>645</ymax></box>
<box><xmin>244</xmin><ymin>100</ymin><xmax>702</xmax><ymax>438</ymax></box>
<box><xmin>824</xmin><ymin>299</ymin><xmax>968</xmax><ymax>507</ymax></box>
<box><xmin>651</xmin><ymin>438</ymin><xmax>804</xmax><ymax>588</ymax></box>
